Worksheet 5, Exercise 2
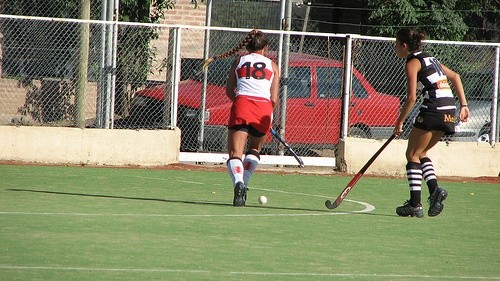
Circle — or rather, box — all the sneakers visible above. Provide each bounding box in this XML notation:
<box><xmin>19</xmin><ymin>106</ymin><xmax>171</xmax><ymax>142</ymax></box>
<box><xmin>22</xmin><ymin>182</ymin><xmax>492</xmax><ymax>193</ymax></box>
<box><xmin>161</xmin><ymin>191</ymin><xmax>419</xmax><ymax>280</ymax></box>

<box><xmin>396</xmin><ymin>200</ymin><xmax>424</xmax><ymax>218</ymax></box>
<box><xmin>233</xmin><ymin>181</ymin><xmax>249</xmax><ymax>207</ymax></box>
<box><xmin>428</xmin><ymin>186</ymin><xmax>448</xmax><ymax>217</ymax></box>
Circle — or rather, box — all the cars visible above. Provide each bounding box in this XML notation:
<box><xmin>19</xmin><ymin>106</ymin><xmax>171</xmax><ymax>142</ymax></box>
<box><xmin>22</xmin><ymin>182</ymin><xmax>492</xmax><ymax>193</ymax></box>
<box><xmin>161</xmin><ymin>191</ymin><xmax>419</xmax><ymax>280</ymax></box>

<box><xmin>128</xmin><ymin>50</ymin><xmax>400</xmax><ymax>151</ymax></box>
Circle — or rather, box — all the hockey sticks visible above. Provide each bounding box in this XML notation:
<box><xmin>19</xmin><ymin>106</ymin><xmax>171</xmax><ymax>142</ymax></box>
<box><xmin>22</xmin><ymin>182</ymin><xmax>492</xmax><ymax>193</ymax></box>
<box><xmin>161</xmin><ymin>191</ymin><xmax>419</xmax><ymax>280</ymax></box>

<box><xmin>325</xmin><ymin>126</ymin><xmax>405</xmax><ymax>209</ymax></box>
<box><xmin>270</xmin><ymin>128</ymin><xmax>304</xmax><ymax>169</ymax></box>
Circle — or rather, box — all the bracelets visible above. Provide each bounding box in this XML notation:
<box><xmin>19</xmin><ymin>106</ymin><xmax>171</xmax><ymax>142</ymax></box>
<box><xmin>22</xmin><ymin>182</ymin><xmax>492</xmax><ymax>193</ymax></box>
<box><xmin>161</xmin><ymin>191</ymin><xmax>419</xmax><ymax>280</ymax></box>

<box><xmin>460</xmin><ymin>104</ymin><xmax>468</xmax><ymax>109</ymax></box>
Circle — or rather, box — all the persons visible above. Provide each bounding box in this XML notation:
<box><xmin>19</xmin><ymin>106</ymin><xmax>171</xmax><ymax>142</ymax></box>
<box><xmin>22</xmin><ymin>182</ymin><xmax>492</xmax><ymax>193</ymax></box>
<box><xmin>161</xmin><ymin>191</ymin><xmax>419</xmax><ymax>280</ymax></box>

<box><xmin>202</xmin><ymin>29</ymin><xmax>279</xmax><ymax>207</ymax></box>
<box><xmin>394</xmin><ymin>28</ymin><xmax>469</xmax><ymax>218</ymax></box>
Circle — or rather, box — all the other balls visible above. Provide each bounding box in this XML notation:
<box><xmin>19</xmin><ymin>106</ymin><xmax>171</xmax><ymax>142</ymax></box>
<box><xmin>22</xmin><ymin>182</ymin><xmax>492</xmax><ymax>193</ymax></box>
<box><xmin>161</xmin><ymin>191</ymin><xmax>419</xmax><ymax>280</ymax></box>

<box><xmin>258</xmin><ymin>196</ymin><xmax>267</xmax><ymax>204</ymax></box>
